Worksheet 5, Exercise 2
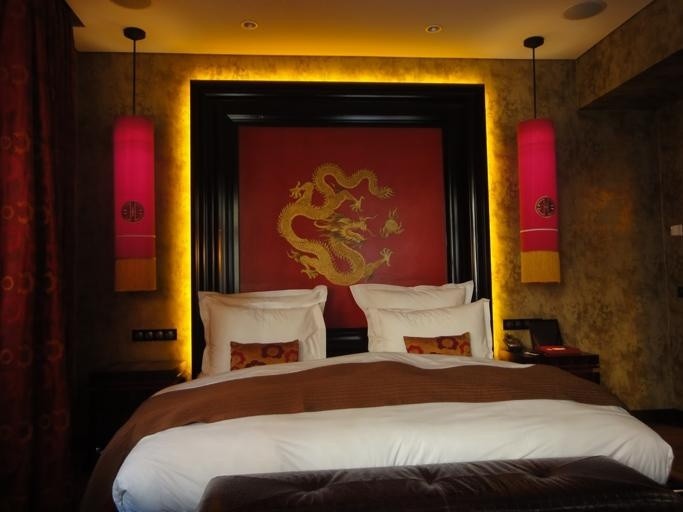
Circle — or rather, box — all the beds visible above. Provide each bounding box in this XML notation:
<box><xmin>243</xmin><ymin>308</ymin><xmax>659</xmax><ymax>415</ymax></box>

<box><xmin>91</xmin><ymin>279</ymin><xmax>678</xmax><ymax>512</ymax></box>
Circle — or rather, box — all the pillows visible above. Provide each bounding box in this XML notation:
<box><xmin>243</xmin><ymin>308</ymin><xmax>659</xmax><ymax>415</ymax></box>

<box><xmin>197</xmin><ymin>279</ymin><xmax>495</xmax><ymax>375</ymax></box>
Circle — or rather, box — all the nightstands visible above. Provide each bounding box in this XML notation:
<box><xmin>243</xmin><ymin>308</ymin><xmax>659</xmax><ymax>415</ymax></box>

<box><xmin>101</xmin><ymin>359</ymin><xmax>186</xmax><ymax>410</ymax></box>
<box><xmin>504</xmin><ymin>318</ymin><xmax>601</xmax><ymax>388</ymax></box>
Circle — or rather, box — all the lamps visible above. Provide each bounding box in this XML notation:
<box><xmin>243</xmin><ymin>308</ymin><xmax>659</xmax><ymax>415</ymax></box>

<box><xmin>517</xmin><ymin>35</ymin><xmax>562</xmax><ymax>284</ymax></box>
<box><xmin>113</xmin><ymin>26</ymin><xmax>158</xmax><ymax>292</ymax></box>
